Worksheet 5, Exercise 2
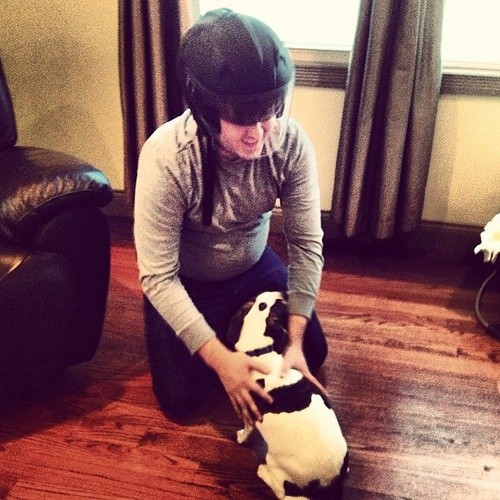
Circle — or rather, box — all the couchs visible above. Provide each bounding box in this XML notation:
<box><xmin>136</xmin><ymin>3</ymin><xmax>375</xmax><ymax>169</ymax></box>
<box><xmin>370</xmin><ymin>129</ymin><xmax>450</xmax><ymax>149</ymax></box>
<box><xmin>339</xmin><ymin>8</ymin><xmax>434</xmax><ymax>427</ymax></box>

<box><xmin>1</xmin><ymin>61</ymin><xmax>114</xmax><ymax>397</ymax></box>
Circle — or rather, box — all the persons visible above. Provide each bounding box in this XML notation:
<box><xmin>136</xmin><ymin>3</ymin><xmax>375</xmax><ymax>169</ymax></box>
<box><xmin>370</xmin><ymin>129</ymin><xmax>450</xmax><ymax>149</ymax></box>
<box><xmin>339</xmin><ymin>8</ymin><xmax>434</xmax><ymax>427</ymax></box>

<box><xmin>134</xmin><ymin>8</ymin><xmax>329</xmax><ymax>419</ymax></box>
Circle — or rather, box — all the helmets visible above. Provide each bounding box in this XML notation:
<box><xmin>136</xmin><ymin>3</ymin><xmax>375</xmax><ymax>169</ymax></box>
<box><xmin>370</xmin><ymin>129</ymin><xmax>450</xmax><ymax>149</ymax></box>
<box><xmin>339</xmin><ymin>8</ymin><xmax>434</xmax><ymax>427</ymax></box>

<box><xmin>177</xmin><ymin>7</ymin><xmax>296</xmax><ymax>131</ymax></box>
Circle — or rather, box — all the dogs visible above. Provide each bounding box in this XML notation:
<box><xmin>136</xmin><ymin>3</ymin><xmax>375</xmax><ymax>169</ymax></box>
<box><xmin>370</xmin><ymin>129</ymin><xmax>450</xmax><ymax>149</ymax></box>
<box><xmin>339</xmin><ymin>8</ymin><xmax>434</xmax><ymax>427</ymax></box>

<box><xmin>225</xmin><ymin>290</ymin><xmax>350</xmax><ymax>500</ymax></box>
